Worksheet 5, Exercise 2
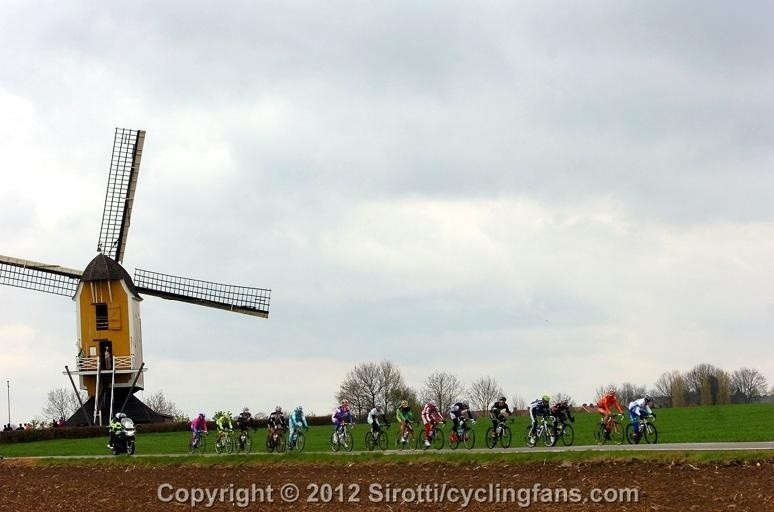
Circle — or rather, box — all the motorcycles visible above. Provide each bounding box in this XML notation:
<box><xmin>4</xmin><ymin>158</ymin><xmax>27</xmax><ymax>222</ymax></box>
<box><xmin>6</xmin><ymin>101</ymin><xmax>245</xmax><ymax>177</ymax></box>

<box><xmin>105</xmin><ymin>416</ymin><xmax>139</xmax><ymax>456</ymax></box>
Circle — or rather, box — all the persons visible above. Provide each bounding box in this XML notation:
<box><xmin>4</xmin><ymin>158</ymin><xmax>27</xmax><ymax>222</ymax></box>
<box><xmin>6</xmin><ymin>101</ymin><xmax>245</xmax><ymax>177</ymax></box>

<box><xmin>106</xmin><ymin>413</ymin><xmax>121</xmax><ymax>447</ymax></box>
<box><xmin>449</xmin><ymin>399</ymin><xmax>475</xmax><ymax>442</ymax></box>
<box><xmin>2</xmin><ymin>417</ymin><xmax>64</xmax><ymax>430</ymax></box>
<box><xmin>77</xmin><ymin>349</ymin><xmax>83</xmax><ymax>370</ymax></box>
<box><xmin>215</xmin><ymin>411</ymin><xmax>233</xmax><ymax>447</ymax></box>
<box><xmin>190</xmin><ymin>412</ymin><xmax>207</xmax><ymax>448</ymax></box>
<box><xmin>330</xmin><ymin>399</ymin><xmax>354</xmax><ymax>448</ymax></box>
<box><xmin>366</xmin><ymin>403</ymin><xmax>387</xmax><ymax>445</ymax></box>
<box><xmin>113</xmin><ymin>412</ymin><xmax>126</xmax><ymax>449</ymax></box>
<box><xmin>104</xmin><ymin>346</ymin><xmax>111</xmax><ymax>369</ymax></box>
<box><xmin>266</xmin><ymin>405</ymin><xmax>287</xmax><ymax>447</ymax></box>
<box><xmin>395</xmin><ymin>399</ymin><xmax>416</xmax><ymax>443</ymax></box>
<box><xmin>627</xmin><ymin>393</ymin><xmax>657</xmax><ymax>441</ymax></box>
<box><xmin>420</xmin><ymin>399</ymin><xmax>444</xmax><ymax>447</ymax></box>
<box><xmin>596</xmin><ymin>389</ymin><xmax>624</xmax><ymax>440</ymax></box>
<box><xmin>527</xmin><ymin>395</ymin><xmax>551</xmax><ymax>444</ymax></box>
<box><xmin>550</xmin><ymin>399</ymin><xmax>575</xmax><ymax>433</ymax></box>
<box><xmin>489</xmin><ymin>396</ymin><xmax>511</xmax><ymax>437</ymax></box>
<box><xmin>236</xmin><ymin>407</ymin><xmax>257</xmax><ymax>451</ymax></box>
<box><xmin>288</xmin><ymin>405</ymin><xmax>307</xmax><ymax>449</ymax></box>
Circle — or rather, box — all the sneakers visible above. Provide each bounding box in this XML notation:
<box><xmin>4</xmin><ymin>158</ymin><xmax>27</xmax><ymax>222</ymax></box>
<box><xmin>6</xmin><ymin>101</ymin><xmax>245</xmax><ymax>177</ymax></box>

<box><xmin>527</xmin><ymin>425</ymin><xmax>568</xmax><ymax>444</ymax></box>
<box><xmin>215</xmin><ymin>442</ymin><xmax>251</xmax><ymax>448</ymax></box>
<box><xmin>601</xmin><ymin>425</ymin><xmax>613</xmax><ymax>441</ymax></box>
<box><xmin>343</xmin><ymin>441</ymin><xmax>349</xmax><ymax>449</ymax></box>
<box><xmin>333</xmin><ymin>432</ymin><xmax>339</xmax><ymax>443</ymax></box>
<box><xmin>491</xmin><ymin>431</ymin><xmax>497</xmax><ymax>438</ymax></box>
<box><xmin>424</xmin><ymin>440</ymin><xmax>432</xmax><ymax>447</ymax></box>
<box><xmin>400</xmin><ymin>436</ymin><xmax>406</xmax><ymax>442</ymax></box>
<box><xmin>265</xmin><ymin>440</ymin><xmax>293</xmax><ymax>451</ymax></box>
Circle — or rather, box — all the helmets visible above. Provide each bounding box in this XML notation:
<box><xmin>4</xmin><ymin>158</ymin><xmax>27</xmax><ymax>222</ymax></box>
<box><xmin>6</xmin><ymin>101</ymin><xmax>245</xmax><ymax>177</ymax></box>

<box><xmin>542</xmin><ymin>395</ymin><xmax>569</xmax><ymax>404</ymax></box>
<box><xmin>242</xmin><ymin>407</ymin><xmax>249</xmax><ymax>413</ymax></box>
<box><xmin>341</xmin><ymin>398</ymin><xmax>349</xmax><ymax>407</ymax></box>
<box><xmin>607</xmin><ymin>388</ymin><xmax>617</xmax><ymax>396</ymax></box>
<box><xmin>223</xmin><ymin>413</ymin><xmax>229</xmax><ymax>419</ymax></box>
<box><xmin>428</xmin><ymin>400</ymin><xmax>437</xmax><ymax>409</ymax></box>
<box><xmin>644</xmin><ymin>393</ymin><xmax>655</xmax><ymax>402</ymax></box>
<box><xmin>295</xmin><ymin>403</ymin><xmax>303</xmax><ymax>412</ymax></box>
<box><xmin>114</xmin><ymin>412</ymin><xmax>128</xmax><ymax>420</ymax></box>
<box><xmin>198</xmin><ymin>412</ymin><xmax>205</xmax><ymax>417</ymax></box>
<box><xmin>498</xmin><ymin>396</ymin><xmax>507</xmax><ymax>403</ymax></box>
<box><xmin>460</xmin><ymin>398</ymin><xmax>470</xmax><ymax>408</ymax></box>
<box><xmin>401</xmin><ymin>400</ymin><xmax>409</xmax><ymax>408</ymax></box>
<box><xmin>375</xmin><ymin>402</ymin><xmax>383</xmax><ymax>410</ymax></box>
<box><xmin>275</xmin><ymin>406</ymin><xmax>283</xmax><ymax>412</ymax></box>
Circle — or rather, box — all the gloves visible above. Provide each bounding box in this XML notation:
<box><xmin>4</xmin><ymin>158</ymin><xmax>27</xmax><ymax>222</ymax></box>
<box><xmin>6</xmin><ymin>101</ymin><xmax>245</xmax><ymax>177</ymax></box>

<box><xmin>430</xmin><ymin>419</ymin><xmax>446</xmax><ymax>425</ymax></box>
<box><xmin>404</xmin><ymin>418</ymin><xmax>417</xmax><ymax>425</ymax></box>
<box><xmin>459</xmin><ymin>419</ymin><xmax>477</xmax><ymax>426</ymax></box>
<box><xmin>639</xmin><ymin>412</ymin><xmax>656</xmax><ymax>420</ymax></box>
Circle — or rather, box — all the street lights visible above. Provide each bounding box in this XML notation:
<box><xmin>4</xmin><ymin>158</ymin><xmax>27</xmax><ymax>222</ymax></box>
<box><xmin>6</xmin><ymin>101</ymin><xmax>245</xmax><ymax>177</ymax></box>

<box><xmin>6</xmin><ymin>380</ymin><xmax>11</xmax><ymax>425</ymax></box>
